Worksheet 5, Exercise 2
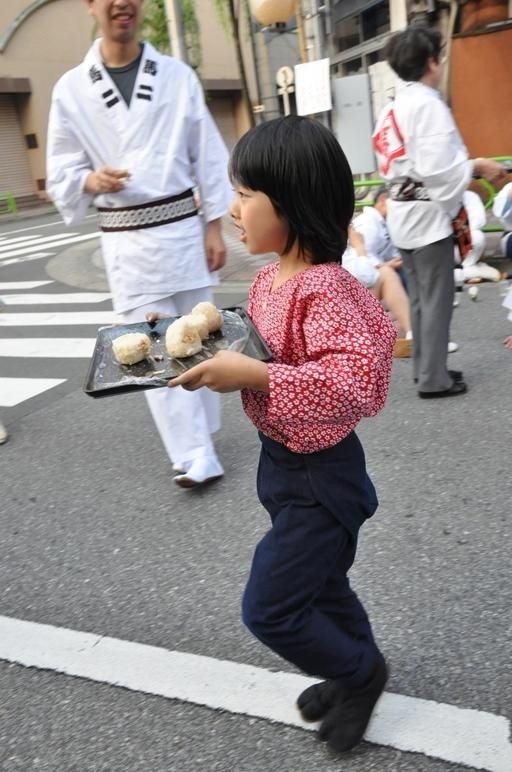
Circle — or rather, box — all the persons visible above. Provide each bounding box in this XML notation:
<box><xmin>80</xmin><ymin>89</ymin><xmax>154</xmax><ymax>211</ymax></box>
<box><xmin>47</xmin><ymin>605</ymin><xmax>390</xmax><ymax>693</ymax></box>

<box><xmin>140</xmin><ymin>114</ymin><xmax>398</xmax><ymax>756</ymax></box>
<box><xmin>341</xmin><ymin>155</ymin><xmax>511</xmax><ymax>368</ymax></box>
<box><xmin>45</xmin><ymin>0</ymin><xmax>235</xmax><ymax>494</ymax></box>
<box><xmin>370</xmin><ymin>30</ymin><xmax>509</xmax><ymax>406</ymax></box>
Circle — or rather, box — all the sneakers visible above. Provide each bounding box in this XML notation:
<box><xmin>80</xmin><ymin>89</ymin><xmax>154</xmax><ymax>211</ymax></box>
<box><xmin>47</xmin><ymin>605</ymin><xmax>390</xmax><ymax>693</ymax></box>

<box><xmin>317</xmin><ymin>657</ymin><xmax>388</xmax><ymax>753</ymax></box>
<box><xmin>477</xmin><ymin>260</ymin><xmax>500</xmax><ymax>281</ymax></box>
<box><xmin>297</xmin><ymin>677</ymin><xmax>340</xmax><ymax>721</ymax></box>
<box><xmin>172</xmin><ymin>459</ymin><xmax>226</xmax><ymax>486</ymax></box>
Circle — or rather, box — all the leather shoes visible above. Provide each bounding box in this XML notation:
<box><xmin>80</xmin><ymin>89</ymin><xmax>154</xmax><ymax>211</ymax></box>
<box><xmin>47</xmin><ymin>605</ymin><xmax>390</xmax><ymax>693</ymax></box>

<box><xmin>415</xmin><ymin>370</ymin><xmax>462</xmax><ymax>381</ymax></box>
<box><xmin>419</xmin><ymin>382</ymin><xmax>466</xmax><ymax>399</ymax></box>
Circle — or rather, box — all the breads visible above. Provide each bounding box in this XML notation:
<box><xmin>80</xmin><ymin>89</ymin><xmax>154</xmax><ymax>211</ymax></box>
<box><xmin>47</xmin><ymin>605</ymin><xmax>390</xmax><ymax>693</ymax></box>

<box><xmin>165</xmin><ymin>319</ymin><xmax>202</xmax><ymax>359</ymax></box>
<box><xmin>111</xmin><ymin>333</ymin><xmax>151</xmax><ymax>364</ymax></box>
<box><xmin>179</xmin><ymin>314</ymin><xmax>209</xmax><ymax>340</ymax></box>
<box><xmin>191</xmin><ymin>301</ymin><xmax>223</xmax><ymax>333</ymax></box>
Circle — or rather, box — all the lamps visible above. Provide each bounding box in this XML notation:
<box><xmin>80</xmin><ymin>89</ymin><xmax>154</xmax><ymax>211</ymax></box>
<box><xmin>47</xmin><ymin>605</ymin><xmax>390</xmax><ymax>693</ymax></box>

<box><xmin>247</xmin><ymin>0</ymin><xmax>298</xmax><ymax>36</ymax></box>
